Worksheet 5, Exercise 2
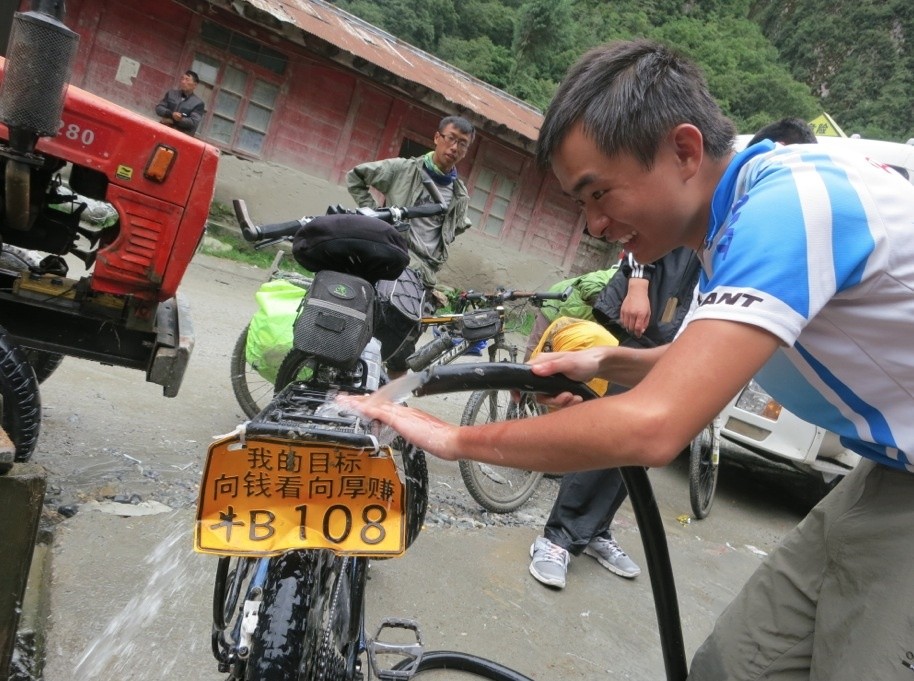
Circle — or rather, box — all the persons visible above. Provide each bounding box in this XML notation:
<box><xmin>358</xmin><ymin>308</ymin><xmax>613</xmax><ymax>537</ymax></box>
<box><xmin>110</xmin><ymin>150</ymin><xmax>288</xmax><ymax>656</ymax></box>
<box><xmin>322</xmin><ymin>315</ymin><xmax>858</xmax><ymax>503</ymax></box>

<box><xmin>333</xmin><ymin>33</ymin><xmax>914</xmax><ymax>681</ymax></box>
<box><xmin>345</xmin><ymin>113</ymin><xmax>478</xmax><ymax>451</ymax></box>
<box><xmin>530</xmin><ymin>115</ymin><xmax>821</xmax><ymax>591</ymax></box>
<box><xmin>152</xmin><ymin>68</ymin><xmax>205</xmax><ymax>136</ymax></box>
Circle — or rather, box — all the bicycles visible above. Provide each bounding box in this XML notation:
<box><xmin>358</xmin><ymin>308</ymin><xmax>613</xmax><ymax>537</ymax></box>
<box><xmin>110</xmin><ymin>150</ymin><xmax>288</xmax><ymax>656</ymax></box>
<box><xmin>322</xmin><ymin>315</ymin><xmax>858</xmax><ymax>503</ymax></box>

<box><xmin>192</xmin><ymin>178</ymin><xmax>455</xmax><ymax>680</ymax></box>
<box><xmin>442</xmin><ymin>288</ymin><xmax>719</xmax><ymax>523</ymax></box>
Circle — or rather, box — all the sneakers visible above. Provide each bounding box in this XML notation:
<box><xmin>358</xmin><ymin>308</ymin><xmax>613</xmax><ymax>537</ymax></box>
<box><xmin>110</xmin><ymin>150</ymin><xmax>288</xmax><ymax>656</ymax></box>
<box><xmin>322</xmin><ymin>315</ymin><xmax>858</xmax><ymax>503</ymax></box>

<box><xmin>529</xmin><ymin>534</ymin><xmax>571</xmax><ymax>589</ymax></box>
<box><xmin>582</xmin><ymin>530</ymin><xmax>642</xmax><ymax>578</ymax></box>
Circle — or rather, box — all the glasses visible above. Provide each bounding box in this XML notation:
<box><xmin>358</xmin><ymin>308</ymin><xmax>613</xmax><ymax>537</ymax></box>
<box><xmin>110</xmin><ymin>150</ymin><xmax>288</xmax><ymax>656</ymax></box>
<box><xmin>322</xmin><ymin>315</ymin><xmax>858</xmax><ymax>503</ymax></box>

<box><xmin>440</xmin><ymin>133</ymin><xmax>470</xmax><ymax>151</ymax></box>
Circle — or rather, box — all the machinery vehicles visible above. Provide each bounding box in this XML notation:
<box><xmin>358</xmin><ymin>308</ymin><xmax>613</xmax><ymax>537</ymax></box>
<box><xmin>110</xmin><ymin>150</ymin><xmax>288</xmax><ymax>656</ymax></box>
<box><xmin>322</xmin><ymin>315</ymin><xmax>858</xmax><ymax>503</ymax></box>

<box><xmin>0</xmin><ymin>9</ymin><xmax>222</xmax><ymax>465</ymax></box>
